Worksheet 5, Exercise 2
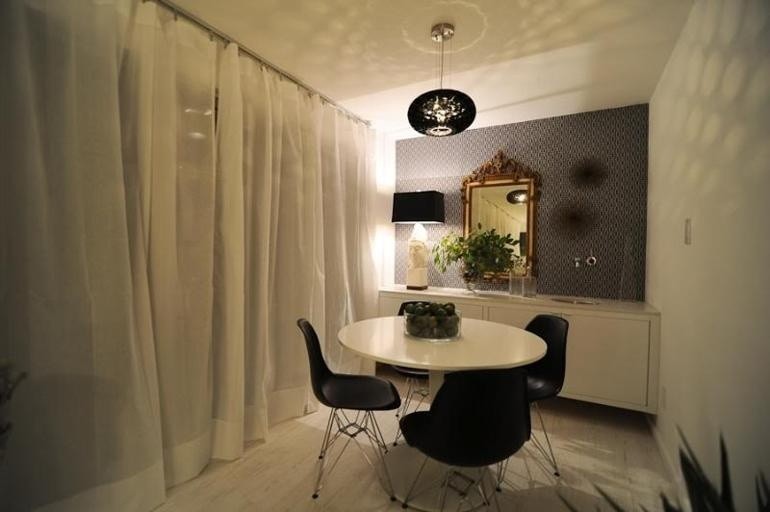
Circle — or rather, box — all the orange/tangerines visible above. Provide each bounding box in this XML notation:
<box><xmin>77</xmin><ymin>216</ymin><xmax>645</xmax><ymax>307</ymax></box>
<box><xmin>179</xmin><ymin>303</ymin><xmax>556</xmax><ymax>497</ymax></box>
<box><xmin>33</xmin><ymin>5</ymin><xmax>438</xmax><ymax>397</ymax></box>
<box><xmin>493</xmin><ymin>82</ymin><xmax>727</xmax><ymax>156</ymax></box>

<box><xmin>406</xmin><ymin>302</ymin><xmax>459</xmax><ymax>338</ymax></box>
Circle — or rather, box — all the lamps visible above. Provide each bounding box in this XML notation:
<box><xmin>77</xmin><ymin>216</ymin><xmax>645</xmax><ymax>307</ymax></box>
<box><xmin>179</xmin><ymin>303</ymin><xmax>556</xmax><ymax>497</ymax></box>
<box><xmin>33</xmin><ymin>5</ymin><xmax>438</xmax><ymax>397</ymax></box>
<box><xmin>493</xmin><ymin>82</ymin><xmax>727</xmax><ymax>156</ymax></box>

<box><xmin>406</xmin><ymin>21</ymin><xmax>477</xmax><ymax>139</ymax></box>
<box><xmin>391</xmin><ymin>189</ymin><xmax>446</xmax><ymax>290</ymax></box>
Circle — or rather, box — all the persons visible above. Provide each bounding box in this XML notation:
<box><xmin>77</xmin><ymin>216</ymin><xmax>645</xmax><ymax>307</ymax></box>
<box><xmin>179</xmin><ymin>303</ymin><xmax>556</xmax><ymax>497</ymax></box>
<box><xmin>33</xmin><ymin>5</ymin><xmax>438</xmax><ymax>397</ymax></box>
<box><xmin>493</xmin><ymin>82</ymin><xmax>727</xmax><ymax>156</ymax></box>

<box><xmin>408</xmin><ymin>237</ymin><xmax>430</xmax><ymax>268</ymax></box>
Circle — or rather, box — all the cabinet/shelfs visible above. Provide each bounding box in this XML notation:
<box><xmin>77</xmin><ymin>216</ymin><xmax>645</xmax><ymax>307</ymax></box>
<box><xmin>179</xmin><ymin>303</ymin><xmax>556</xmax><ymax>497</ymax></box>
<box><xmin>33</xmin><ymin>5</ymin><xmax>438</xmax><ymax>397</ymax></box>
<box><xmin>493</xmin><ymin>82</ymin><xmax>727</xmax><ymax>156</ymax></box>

<box><xmin>488</xmin><ymin>307</ymin><xmax>652</xmax><ymax>409</ymax></box>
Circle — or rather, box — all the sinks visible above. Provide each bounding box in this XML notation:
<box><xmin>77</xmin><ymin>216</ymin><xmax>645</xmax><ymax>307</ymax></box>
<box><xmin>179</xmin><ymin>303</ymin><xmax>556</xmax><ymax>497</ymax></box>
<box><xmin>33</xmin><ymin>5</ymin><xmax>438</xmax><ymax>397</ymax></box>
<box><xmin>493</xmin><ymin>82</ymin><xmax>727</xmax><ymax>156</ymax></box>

<box><xmin>549</xmin><ymin>297</ymin><xmax>600</xmax><ymax>306</ymax></box>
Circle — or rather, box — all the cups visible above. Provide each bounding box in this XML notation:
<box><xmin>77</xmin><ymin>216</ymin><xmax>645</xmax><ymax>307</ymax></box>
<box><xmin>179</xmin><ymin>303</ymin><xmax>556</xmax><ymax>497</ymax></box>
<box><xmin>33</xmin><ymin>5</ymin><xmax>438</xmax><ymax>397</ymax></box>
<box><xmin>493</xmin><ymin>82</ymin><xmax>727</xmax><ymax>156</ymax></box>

<box><xmin>508</xmin><ymin>276</ymin><xmax>537</xmax><ymax>297</ymax></box>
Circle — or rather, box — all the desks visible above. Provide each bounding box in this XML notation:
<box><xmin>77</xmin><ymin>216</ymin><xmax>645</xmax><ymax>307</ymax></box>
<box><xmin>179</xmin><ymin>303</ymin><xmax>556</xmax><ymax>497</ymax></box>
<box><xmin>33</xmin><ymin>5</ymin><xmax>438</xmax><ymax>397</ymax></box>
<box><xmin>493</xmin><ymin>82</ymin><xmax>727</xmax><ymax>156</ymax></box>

<box><xmin>339</xmin><ymin>314</ymin><xmax>548</xmax><ymax>487</ymax></box>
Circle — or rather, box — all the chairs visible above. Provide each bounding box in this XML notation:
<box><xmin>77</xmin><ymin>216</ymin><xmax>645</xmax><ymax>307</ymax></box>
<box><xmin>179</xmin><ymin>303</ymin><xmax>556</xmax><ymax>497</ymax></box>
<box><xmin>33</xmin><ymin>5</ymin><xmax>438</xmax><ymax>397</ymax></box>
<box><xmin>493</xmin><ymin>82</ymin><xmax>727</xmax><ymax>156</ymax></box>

<box><xmin>398</xmin><ymin>368</ymin><xmax>532</xmax><ymax>511</ymax></box>
<box><xmin>295</xmin><ymin>316</ymin><xmax>401</xmax><ymax>502</ymax></box>
<box><xmin>497</xmin><ymin>312</ymin><xmax>570</xmax><ymax>477</ymax></box>
<box><xmin>391</xmin><ymin>363</ymin><xmax>444</xmax><ymax>447</ymax></box>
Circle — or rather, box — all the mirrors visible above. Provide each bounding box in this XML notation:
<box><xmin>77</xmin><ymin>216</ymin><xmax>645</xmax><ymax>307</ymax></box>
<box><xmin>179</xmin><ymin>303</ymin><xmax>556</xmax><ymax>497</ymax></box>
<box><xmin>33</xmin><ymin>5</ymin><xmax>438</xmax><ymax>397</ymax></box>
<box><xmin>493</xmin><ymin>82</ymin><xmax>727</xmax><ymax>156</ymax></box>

<box><xmin>457</xmin><ymin>150</ymin><xmax>542</xmax><ymax>282</ymax></box>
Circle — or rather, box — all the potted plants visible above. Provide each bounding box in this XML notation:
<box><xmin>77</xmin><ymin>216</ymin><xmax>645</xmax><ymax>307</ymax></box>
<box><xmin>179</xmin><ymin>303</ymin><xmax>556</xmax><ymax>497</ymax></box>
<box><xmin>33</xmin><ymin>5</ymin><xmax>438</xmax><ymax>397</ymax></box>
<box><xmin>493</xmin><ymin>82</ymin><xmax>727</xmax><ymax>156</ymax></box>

<box><xmin>429</xmin><ymin>230</ymin><xmax>521</xmax><ymax>297</ymax></box>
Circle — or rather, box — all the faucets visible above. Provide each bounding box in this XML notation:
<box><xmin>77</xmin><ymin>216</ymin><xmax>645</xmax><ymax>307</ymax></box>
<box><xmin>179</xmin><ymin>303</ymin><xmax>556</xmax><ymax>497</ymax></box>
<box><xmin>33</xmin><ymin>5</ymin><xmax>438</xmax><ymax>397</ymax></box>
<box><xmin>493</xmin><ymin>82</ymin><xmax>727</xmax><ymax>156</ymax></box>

<box><xmin>574</xmin><ymin>257</ymin><xmax>581</xmax><ymax>271</ymax></box>
